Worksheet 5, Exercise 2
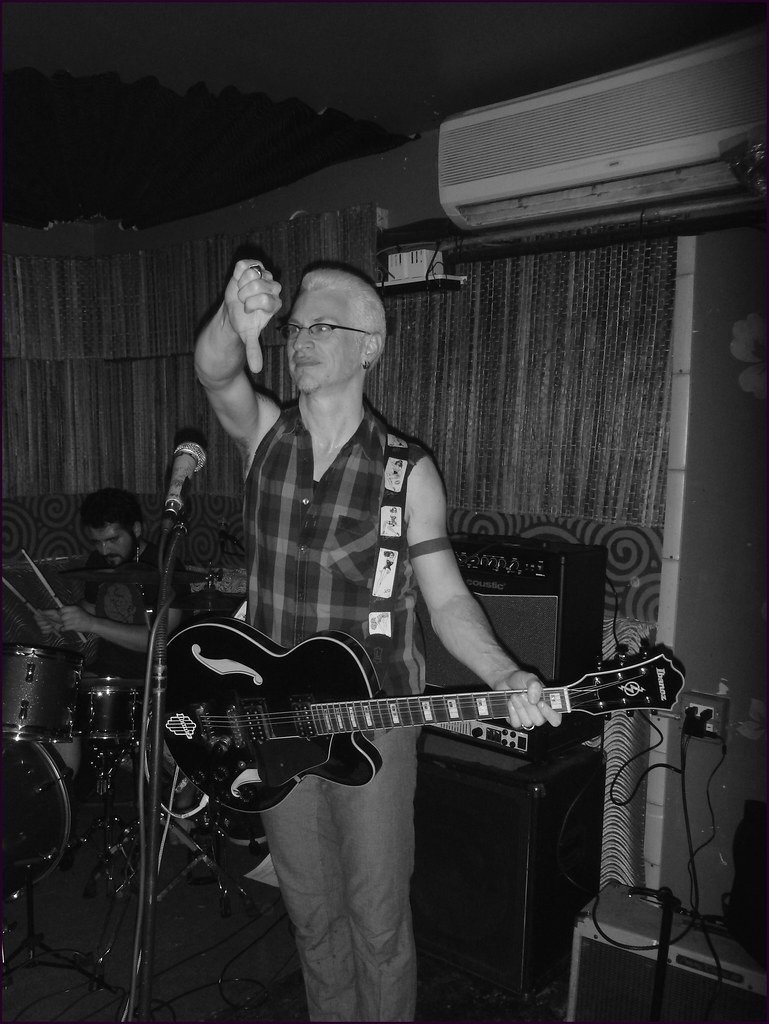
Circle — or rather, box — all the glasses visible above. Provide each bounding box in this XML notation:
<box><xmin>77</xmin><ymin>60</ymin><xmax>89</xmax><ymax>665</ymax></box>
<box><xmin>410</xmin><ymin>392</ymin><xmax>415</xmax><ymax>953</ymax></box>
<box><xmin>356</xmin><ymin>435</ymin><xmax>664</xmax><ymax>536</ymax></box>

<box><xmin>275</xmin><ymin>323</ymin><xmax>372</xmax><ymax>340</ymax></box>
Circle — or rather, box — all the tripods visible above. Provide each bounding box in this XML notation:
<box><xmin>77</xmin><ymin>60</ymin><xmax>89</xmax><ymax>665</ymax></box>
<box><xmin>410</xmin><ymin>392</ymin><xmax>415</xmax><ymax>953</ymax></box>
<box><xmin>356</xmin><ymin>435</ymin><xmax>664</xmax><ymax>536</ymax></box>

<box><xmin>0</xmin><ymin>751</ymin><xmax>260</xmax><ymax>994</ymax></box>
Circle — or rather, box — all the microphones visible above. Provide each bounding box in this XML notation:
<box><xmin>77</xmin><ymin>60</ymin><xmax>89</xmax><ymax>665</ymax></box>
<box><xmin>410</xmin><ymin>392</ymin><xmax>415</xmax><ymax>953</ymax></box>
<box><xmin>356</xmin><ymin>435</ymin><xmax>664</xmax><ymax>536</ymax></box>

<box><xmin>163</xmin><ymin>440</ymin><xmax>208</xmax><ymax>532</ymax></box>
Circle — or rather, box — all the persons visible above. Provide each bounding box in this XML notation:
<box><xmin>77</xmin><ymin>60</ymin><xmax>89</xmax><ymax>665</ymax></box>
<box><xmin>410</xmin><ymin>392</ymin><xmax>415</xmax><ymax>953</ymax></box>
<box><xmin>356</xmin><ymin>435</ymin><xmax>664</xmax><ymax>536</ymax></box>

<box><xmin>195</xmin><ymin>257</ymin><xmax>561</xmax><ymax>1022</ymax></box>
<box><xmin>34</xmin><ymin>488</ymin><xmax>195</xmax><ymax>845</ymax></box>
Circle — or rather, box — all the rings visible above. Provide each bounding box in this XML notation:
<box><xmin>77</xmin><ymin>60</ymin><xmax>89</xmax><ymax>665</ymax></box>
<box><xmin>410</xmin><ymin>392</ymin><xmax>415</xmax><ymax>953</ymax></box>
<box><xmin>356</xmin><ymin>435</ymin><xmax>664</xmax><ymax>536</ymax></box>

<box><xmin>521</xmin><ymin>724</ymin><xmax>535</xmax><ymax>731</ymax></box>
<box><xmin>249</xmin><ymin>264</ymin><xmax>262</xmax><ymax>278</ymax></box>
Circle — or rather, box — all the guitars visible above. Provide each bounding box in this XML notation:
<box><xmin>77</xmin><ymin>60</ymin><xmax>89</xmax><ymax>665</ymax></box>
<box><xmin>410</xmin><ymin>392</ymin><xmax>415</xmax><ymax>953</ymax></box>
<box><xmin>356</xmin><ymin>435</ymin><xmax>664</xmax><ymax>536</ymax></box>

<box><xmin>154</xmin><ymin>617</ymin><xmax>684</xmax><ymax>812</ymax></box>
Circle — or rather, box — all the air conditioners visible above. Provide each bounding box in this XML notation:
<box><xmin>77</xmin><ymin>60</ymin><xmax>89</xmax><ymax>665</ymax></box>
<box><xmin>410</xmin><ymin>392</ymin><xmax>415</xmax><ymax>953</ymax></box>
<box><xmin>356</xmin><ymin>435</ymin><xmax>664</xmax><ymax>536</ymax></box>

<box><xmin>437</xmin><ymin>24</ymin><xmax>769</xmax><ymax>230</ymax></box>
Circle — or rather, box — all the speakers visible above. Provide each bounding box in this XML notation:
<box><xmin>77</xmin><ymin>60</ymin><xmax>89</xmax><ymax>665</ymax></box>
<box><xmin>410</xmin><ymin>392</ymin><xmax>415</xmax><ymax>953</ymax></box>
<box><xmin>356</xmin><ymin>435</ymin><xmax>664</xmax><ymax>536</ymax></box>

<box><xmin>408</xmin><ymin>731</ymin><xmax>609</xmax><ymax>994</ymax></box>
<box><xmin>566</xmin><ymin>878</ymin><xmax>768</xmax><ymax>1023</ymax></box>
<box><xmin>417</xmin><ymin>532</ymin><xmax>607</xmax><ymax>763</ymax></box>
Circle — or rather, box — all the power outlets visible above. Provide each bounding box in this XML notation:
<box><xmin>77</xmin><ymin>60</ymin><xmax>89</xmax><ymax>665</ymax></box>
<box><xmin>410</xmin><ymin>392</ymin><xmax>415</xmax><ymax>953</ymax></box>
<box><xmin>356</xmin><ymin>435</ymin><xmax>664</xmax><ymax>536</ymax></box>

<box><xmin>681</xmin><ymin>691</ymin><xmax>731</xmax><ymax>747</ymax></box>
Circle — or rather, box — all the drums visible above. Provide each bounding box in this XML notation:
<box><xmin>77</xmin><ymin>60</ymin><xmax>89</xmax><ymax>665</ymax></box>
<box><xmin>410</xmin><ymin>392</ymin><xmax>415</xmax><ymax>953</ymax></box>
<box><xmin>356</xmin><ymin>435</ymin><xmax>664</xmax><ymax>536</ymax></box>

<box><xmin>1</xmin><ymin>740</ymin><xmax>75</xmax><ymax>898</ymax></box>
<box><xmin>81</xmin><ymin>677</ymin><xmax>146</xmax><ymax>738</ymax></box>
<box><xmin>1</xmin><ymin>648</ymin><xmax>79</xmax><ymax>740</ymax></box>
